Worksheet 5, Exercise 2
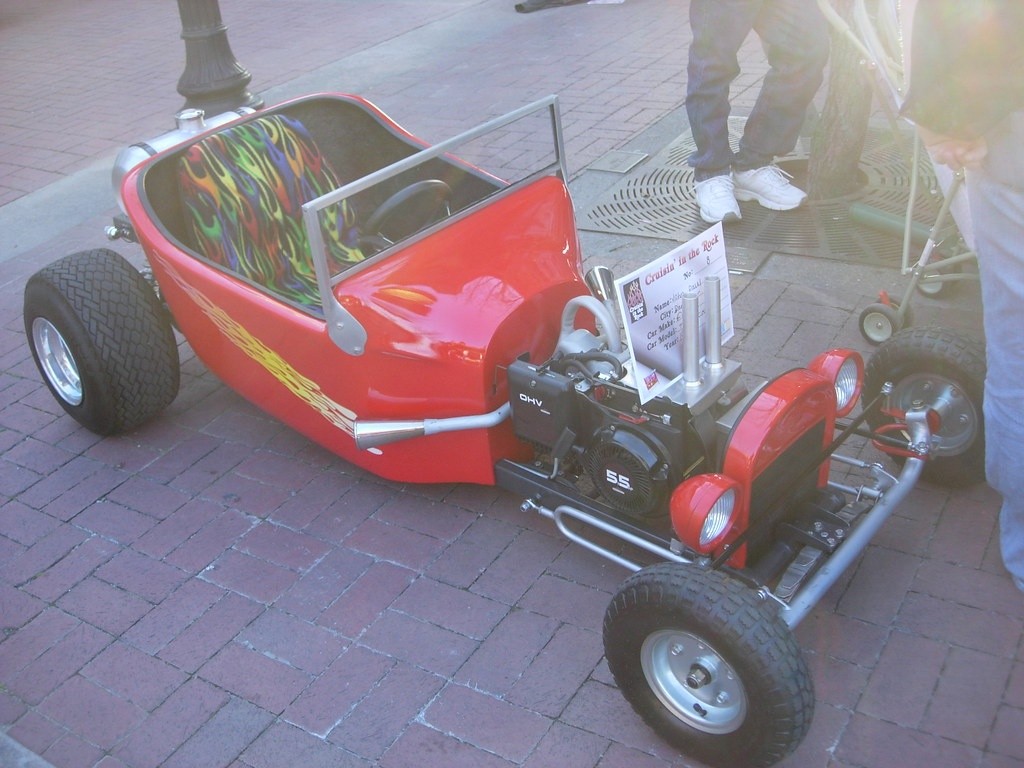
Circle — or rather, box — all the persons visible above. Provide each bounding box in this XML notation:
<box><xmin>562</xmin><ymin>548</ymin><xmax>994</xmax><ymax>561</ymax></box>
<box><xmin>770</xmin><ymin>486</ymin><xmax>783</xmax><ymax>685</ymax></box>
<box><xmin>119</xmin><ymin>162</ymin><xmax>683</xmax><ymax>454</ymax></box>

<box><xmin>896</xmin><ymin>0</ymin><xmax>1024</xmax><ymax>589</ymax></box>
<box><xmin>686</xmin><ymin>0</ymin><xmax>830</xmax><ymax>224</ymax></box>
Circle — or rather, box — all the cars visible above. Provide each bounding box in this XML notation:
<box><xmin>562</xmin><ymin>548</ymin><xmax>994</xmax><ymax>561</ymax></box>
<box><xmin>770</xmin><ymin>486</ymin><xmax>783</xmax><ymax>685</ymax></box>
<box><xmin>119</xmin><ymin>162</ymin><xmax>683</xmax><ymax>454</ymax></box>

<box><xmin>22</xmin><ymin>92</ymin><xmax>988</xmax><ymax>767</ymax></box>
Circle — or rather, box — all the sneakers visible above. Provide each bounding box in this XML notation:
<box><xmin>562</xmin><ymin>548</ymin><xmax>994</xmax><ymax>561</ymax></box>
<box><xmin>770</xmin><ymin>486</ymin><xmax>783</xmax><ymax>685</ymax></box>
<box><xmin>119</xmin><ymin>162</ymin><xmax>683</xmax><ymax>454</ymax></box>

<box><xmin>729</xmin><ymin>159</ymin><xmax>808</xmax><ymax>211</ymax></box>
<box><xmin>686</xmin><ymin>170</ymin><xmax>742</xmax><ymax>223</ymax></box>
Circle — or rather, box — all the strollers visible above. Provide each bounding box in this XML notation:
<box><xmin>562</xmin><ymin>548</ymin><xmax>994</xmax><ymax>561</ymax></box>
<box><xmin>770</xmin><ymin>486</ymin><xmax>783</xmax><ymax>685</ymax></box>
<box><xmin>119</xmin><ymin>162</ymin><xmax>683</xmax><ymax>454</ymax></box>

<box><xmin>819</xmin><ymin>0</ymin><xmax>979</xmax><ymax>346</ymax></box>
